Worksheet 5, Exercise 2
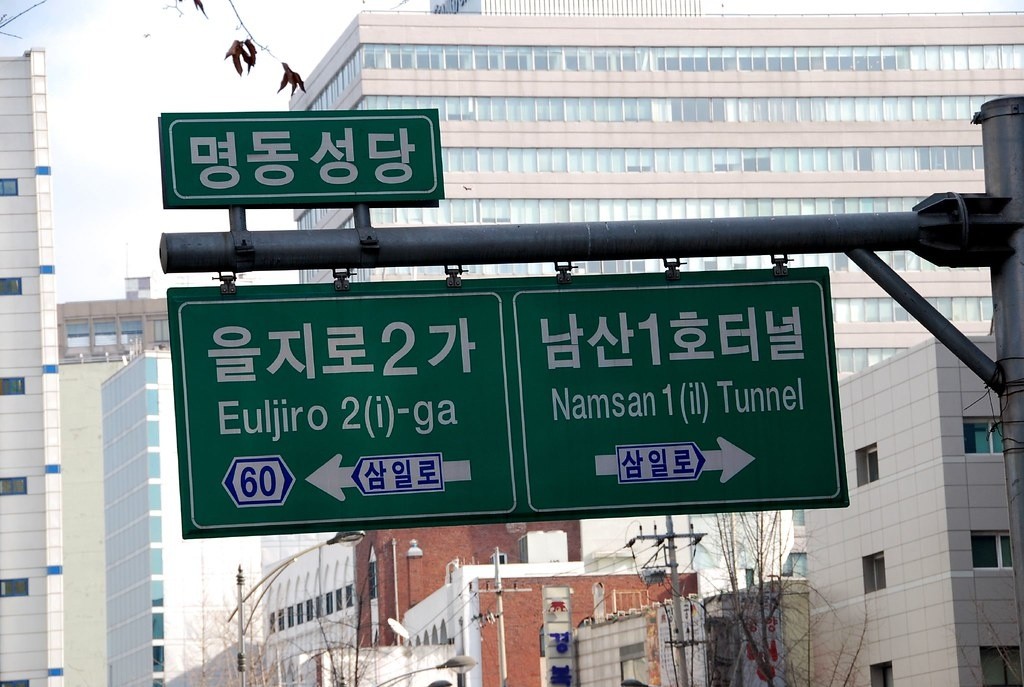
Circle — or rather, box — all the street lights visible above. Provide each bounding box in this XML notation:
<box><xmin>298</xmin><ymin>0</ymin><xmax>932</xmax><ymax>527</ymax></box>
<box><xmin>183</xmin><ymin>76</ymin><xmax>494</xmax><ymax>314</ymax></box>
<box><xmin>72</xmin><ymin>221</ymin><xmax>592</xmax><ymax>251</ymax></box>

<box><xmin>375</xmin><ymin>654</ymin><xmax>479</xmax><ymax>686</ymax></box>
<box><xmin>227</xmin><ymin>530</ymin><xmax>367</xmax><ymax>687</ymax></box>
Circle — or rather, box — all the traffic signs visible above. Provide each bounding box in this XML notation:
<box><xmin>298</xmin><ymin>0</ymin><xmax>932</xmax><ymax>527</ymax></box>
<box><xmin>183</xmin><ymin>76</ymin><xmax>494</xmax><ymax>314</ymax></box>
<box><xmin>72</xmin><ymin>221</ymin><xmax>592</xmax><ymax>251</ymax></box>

<box><xmin>166</xmin><ymin>265</ymin><xmax>852</xmax><ymax>541</ymax></box>
